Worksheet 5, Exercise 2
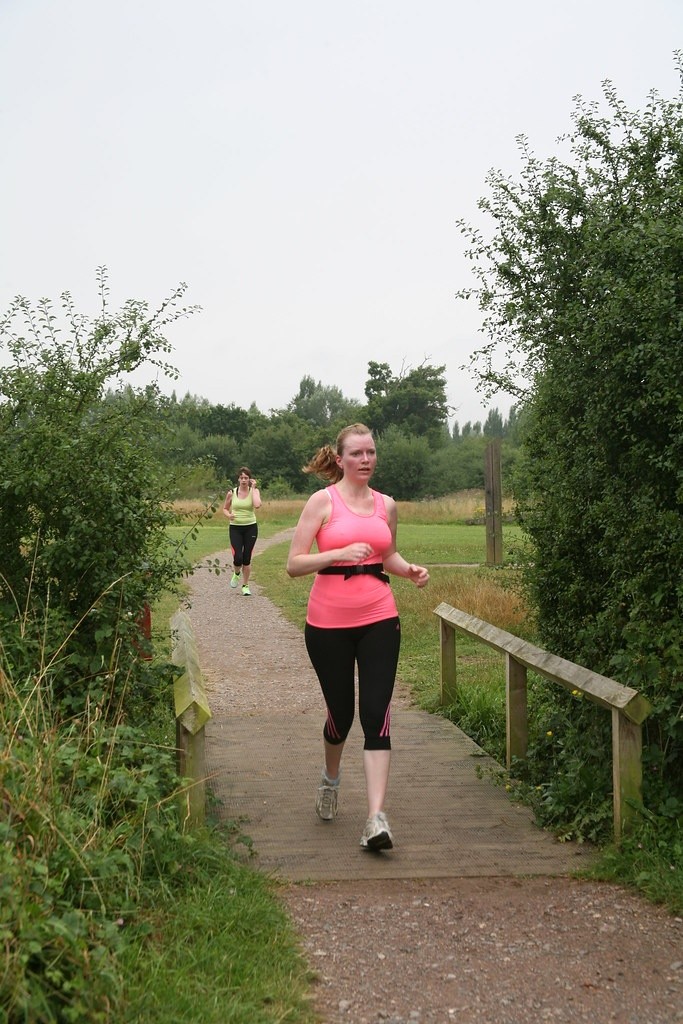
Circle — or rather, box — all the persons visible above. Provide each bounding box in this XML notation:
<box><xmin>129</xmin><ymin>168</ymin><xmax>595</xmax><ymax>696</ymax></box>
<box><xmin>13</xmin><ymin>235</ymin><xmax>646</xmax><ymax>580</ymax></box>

<box><xmin>282</xmin><ymin>424</ymin><xmax>430</xmax><ymax>852</ymax></box>
<box><xmin>222</xmin><ymin>467</ymin><xmax>264</xmax><ymax>596</ymax></box>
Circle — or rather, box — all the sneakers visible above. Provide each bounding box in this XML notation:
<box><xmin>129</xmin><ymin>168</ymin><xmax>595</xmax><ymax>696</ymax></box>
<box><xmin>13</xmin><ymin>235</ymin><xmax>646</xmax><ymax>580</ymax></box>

<box><xmin>315</xmin><ymin>763</ymin><xmax>342</xmax><ymax>820</ymax></box>
<box><xmin>230</xmin><ymin>571</ymin><xmax>251</xmax><ymax>595</ymax></box>
<box><xmin>359</xmin><ymin>811</ymin><xmax>394</xmax><ymax>851</ymax></box>
